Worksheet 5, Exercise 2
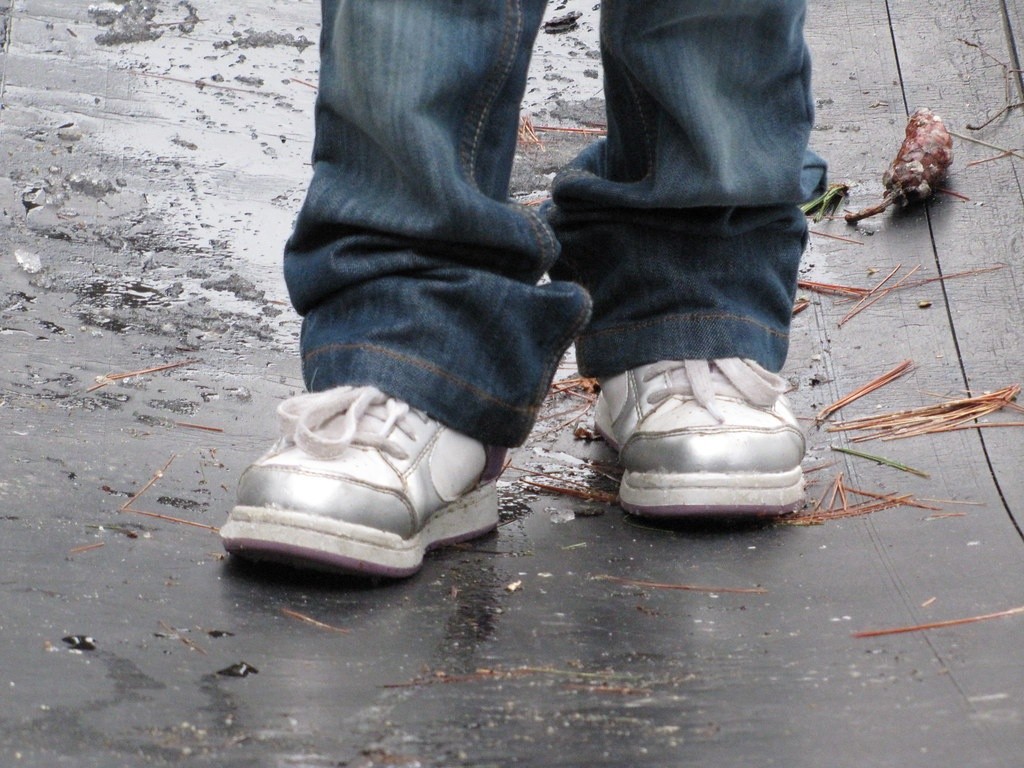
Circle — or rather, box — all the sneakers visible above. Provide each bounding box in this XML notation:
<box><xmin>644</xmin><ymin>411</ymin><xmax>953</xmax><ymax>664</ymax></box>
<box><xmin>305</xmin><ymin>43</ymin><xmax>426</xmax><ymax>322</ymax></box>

<box><xmin>591</xmin><ymin>347</ymin><xmax>807</xmax><ymax>526</ymax></box>
<box><xmin>216</xmin><ymin>385</ymin><xmax>509</xmax><ymax>582</ymax></box>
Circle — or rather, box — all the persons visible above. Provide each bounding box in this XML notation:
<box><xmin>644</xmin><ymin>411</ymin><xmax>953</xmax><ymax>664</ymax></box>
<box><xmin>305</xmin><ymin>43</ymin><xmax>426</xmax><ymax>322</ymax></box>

<box><xmin>220</xmin><ymin>0</ymin><xmax>828</xmax><ymax>575</ymax></box>
